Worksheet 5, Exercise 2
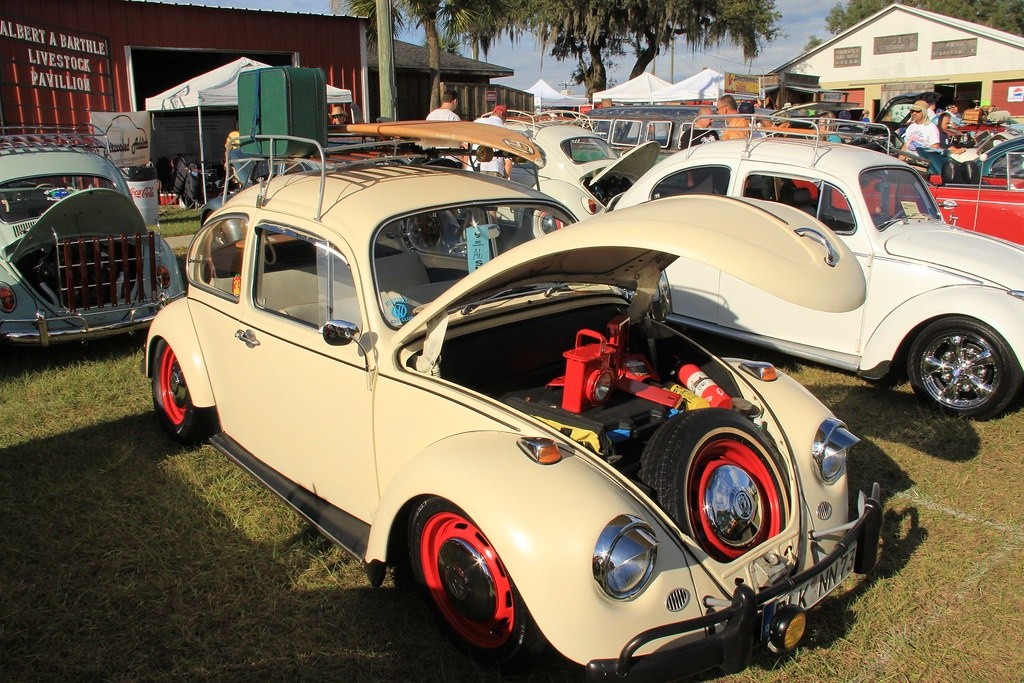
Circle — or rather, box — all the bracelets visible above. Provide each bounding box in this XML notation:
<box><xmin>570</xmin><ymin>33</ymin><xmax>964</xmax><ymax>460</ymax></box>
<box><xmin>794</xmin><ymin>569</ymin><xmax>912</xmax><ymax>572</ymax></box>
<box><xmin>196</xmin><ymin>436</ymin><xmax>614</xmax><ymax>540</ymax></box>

<box><xmin>505</xmin><ymin>176</ymin><xmax>510</xmax><ymax>180</ymax></box>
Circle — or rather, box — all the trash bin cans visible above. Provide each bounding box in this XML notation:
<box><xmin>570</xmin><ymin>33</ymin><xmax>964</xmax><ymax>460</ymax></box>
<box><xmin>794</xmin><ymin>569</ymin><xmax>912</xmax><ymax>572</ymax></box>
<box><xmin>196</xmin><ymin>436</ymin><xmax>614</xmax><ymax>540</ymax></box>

<box><xmin>119</xmin><ymin>165</ymin><xmax>159</xmax><ymax>224</ymax></box>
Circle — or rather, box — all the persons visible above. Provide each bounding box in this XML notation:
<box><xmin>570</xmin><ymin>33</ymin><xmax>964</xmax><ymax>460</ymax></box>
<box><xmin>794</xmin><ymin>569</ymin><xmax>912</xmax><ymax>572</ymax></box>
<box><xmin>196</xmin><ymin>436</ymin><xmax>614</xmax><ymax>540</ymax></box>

<box><xmin>224</xmin><ymin>120</ymin><xmax>240</xmax><ymax>188</ymax></box>
<box><xmin>680</xmin><ymin>94</ymin><xmax>967</xmax><ymax>187</ymax></box>
<box><xmin>426</xmin><ymin>89</ymin><xmax>512</xmax><ymax>224</ymax></box>
<box><xmin>328</xmin><ymin>107</ymin><xmax>350</xmax><ymax>124</ymax></box>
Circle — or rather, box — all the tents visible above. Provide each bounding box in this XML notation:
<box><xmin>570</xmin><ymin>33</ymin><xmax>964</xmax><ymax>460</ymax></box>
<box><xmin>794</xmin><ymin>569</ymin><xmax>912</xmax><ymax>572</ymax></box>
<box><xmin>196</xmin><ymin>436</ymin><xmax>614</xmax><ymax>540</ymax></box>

<box><xmin>592</xmin><ymin>68</ymin><xmax>766</xmax><ymax>110</ymax></box>
<box><xmin>523</xmin><ymin>79</ymin><xmax>589</xmax><ymax>113</ymax></box>
<box><xmin>146</xmin><ymin>57</ymin><xmax>353</xmax><ymax>205</ymax></box>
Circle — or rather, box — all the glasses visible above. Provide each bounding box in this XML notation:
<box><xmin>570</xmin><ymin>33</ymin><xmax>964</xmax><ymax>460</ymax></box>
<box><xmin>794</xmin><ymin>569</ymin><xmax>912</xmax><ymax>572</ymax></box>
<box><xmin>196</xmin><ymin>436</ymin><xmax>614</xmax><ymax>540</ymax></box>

<box><xmin>717</xmin><ymin>104</ymin><xmax>729</xmax><ymax>111</ymax></box>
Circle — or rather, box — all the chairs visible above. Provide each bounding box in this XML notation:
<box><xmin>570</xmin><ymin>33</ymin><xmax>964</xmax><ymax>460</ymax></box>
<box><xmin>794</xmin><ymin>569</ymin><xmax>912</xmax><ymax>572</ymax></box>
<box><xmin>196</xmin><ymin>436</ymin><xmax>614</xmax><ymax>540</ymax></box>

<box><xmin>792</xmin><ymin>187</ymin><xmax>817</xmax><ymax>217</ymax></box>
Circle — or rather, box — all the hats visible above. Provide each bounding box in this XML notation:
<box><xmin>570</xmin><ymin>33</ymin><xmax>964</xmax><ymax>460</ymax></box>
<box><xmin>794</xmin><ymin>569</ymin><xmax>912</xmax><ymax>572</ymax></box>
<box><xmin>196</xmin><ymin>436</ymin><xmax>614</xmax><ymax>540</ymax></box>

<box><xmin>782</xmin><ymin>101</ymin><xmax>793</xmax><ymax>109</ymax></box>
<box><xmin>491</xmin><ymin>104</ymin><xmax>507</xmax><ymax>118</ymax></box>
<box><xmin>909</xmin><ymin>98</ymin><xmax>931</xmax><ymax>120</ymax></box>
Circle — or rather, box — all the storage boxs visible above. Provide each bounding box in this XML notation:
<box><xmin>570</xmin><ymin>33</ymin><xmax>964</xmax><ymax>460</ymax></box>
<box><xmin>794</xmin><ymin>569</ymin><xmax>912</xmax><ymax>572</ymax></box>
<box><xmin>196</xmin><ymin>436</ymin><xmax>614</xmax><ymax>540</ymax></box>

<box><xmin>160</xmin><ymin>193</ymin><xmax>179</xmax><ymax>205</ymax></box>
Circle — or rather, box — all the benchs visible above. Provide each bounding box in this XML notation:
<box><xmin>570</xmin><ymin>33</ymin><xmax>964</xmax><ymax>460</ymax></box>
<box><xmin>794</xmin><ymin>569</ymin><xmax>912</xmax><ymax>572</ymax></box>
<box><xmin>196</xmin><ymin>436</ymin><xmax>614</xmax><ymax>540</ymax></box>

<box><xmin>207</xmin><ymin>250</ymin><xmax>430</xmax><ymax>310</ymax></box>
<box><xmin>277</xmin><ymin>277</ymin><xmax>461</xmax><ymax>331</ymax></box>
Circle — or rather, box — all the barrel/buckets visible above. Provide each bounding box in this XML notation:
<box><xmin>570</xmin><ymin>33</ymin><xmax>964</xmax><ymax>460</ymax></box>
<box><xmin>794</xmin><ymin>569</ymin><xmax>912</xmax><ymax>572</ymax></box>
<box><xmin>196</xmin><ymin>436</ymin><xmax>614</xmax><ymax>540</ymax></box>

<box><xmin>125</xmin><ymin>178</ymin><xmax>160</xmax><ymax>226</ymax></box>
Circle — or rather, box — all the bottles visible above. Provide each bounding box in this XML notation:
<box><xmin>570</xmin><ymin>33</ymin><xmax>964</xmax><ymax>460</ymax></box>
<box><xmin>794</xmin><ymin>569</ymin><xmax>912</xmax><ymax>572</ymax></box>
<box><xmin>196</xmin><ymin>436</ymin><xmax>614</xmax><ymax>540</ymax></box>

<box><xmin>666</xmin><ymin>353</ymin><xmax>734</xmax><ymax>411</ymax></box>
<box><xmin>642</xmin><ymin>377</ymin><xmax>711</xmax><ymax>410</ymax></box>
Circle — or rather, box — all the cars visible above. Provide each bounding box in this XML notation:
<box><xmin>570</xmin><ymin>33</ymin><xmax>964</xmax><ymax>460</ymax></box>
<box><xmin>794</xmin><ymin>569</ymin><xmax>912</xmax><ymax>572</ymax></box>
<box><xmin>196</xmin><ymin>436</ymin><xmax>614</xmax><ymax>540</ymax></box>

<box><xmin>140</xmin><ymin>133</ymin><xmax>880</xmax><ymax>683</ymax></box>
<box><xmin>0</xmin><ymin>123</ymin><xmax>189</xmax><ymax>353</ymax></box>
<box><xmin>597</xmin><ymin>111</ymin><xmax>1024</xmax><ymax>420</ymax></box>
<box><xmin>324</xmin><ymin>95</ymin><xmax>1024</xmax><ymax>276</ymax></box>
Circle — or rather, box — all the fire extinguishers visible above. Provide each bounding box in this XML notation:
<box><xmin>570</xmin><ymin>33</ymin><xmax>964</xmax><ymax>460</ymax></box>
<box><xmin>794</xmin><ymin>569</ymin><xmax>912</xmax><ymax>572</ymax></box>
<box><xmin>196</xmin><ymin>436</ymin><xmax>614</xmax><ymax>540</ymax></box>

<box><xmin>665</xmin><ymin>357</ymin><xmax>733</xmax><ymax>410</ymax></box>
